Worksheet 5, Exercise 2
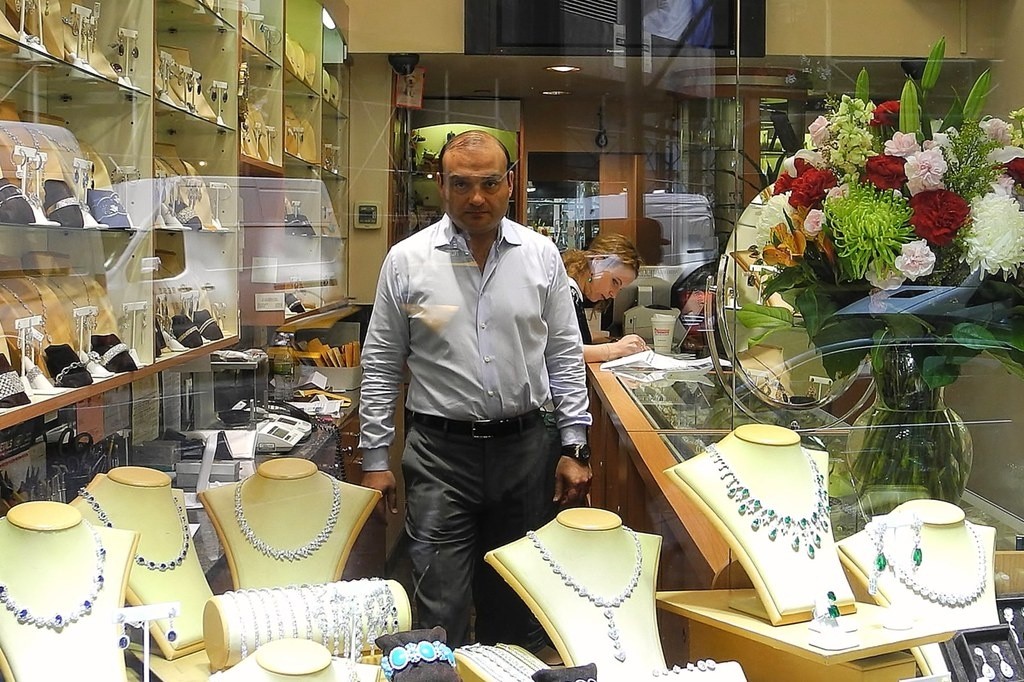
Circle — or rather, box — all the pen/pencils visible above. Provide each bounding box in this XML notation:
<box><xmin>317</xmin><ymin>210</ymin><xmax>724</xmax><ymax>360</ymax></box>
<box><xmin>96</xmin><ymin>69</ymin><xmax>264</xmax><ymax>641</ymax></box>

<box><xmin>605</xmin><ymin>336</ymin><xmax>622</xmax><ymax>342</ymax></box>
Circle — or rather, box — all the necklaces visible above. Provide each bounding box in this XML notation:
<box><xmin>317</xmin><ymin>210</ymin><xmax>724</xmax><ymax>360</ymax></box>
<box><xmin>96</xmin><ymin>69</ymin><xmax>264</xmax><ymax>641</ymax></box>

<box><xmin>0</xmin><ymin>515</ymin><xmax>107</xmax><ymax>631</ymax></box>
<box><xmin>864</xmin><ymin>511</ymin><xmax>988</xmax><ymax>609</ymax></box>
<box><xmin>77</xmin><ymin>478</ymin><xmax>190</xmax><ymax>573</ymax></box>
<box><xmin>525</xmin><ymin>524</ymin><xmax>644</xmax><ymax>663</ymax></box>
<box><xmin>701</xmin><ymin>442</ymin><xmax>833</xmax><ymax>560</ymax></box>
<box><xmin>233</xmin><ymin>470</ymin><xmax>342</xmax><ymax>563</ymax></box>
<box><xmin>0</xmin><ymin>0</ymin><xmax>347</xmax><ymax>349</ymax></box>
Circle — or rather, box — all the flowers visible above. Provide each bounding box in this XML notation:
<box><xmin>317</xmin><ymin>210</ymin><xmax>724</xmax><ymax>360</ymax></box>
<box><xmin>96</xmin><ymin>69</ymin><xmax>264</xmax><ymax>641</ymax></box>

<box><xmin>736</xmin><ymin>35</ymin><xmax>1024</xmax><ymax>392</ymax></box>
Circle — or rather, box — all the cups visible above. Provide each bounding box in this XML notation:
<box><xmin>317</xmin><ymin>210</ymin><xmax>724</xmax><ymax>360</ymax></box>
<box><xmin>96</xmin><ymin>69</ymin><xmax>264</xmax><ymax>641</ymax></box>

<box><xmin>651</xmin><ymin>313</ymin><xmax>677</xmax><ymax>354</ymax></box>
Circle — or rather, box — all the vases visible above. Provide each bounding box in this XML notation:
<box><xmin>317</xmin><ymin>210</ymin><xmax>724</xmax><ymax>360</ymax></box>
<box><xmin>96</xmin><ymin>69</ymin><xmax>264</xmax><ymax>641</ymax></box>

<box><xmin>845</xmin><ymin>345</ymin><xmax>973</xmax><ymax>516</ymax></box>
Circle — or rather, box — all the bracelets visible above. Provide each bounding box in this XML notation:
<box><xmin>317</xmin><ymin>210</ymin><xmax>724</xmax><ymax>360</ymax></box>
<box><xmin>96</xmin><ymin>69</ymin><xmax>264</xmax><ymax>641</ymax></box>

<box><xmin>0</xmin><ymin>370</ymin><xmax>26</xmax><ymax>400</ymax></box>
<box><xmin>605</xmin><ymin>343</ymin><xmax>611</xmax><ymax>362</ymax></box>
<box><xmin>380</xmin><ymin>640</ymin><xmax>457</xmax><ymax>682</ymax></box>
<box><xmin>54</xmin><ymin>351</ymin><xmax>101</xmax><ymax>387</ymax></box>
<box><xmin>26</xmin><ymin>365</ymin><xmax>44</xmax><ymax>385</ymax></box>
<box><xmin>100</xmin><ymin>342</ymin><xmax>130</xmax><ymax>367</ymax></box>
<box><xmin>218</xmin><ymin>576</ymin><xmax>399</xmax><ymax>664</ymax></box>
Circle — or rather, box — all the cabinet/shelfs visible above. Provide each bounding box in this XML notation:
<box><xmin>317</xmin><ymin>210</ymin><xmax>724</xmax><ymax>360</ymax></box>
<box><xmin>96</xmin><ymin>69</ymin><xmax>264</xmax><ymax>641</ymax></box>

<box><xmin>0</xmin><ymin>0</ymin><xmax>406</xmax><ymax>595</ymax></box>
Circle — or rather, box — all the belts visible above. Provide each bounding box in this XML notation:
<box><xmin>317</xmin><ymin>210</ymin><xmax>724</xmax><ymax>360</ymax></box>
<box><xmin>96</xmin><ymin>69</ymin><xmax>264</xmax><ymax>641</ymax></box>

<box><xmin>413</xmin><ymin>411</ymin><xmax>541</xmax><ymax>439</ymax></box>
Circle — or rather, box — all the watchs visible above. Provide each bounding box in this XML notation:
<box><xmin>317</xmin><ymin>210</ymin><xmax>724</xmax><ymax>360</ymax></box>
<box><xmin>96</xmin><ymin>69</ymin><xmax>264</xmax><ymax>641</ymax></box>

<box><xmin>561</xmin><ymin>443</ymin><xmax>592</xmax><ymax>462</ymax></box>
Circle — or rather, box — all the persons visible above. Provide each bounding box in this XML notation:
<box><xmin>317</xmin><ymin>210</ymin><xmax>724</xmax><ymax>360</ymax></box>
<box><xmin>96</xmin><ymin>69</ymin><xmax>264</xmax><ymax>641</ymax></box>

<box><xmin>357</xmin><ymin>129</ymin><xmax>594</xmax><ymax>666</ymax></box>
<box><xmin>526</xmin><ymin>230</ymin><xmax>652</xmax><ymax>667</ymax></box>
